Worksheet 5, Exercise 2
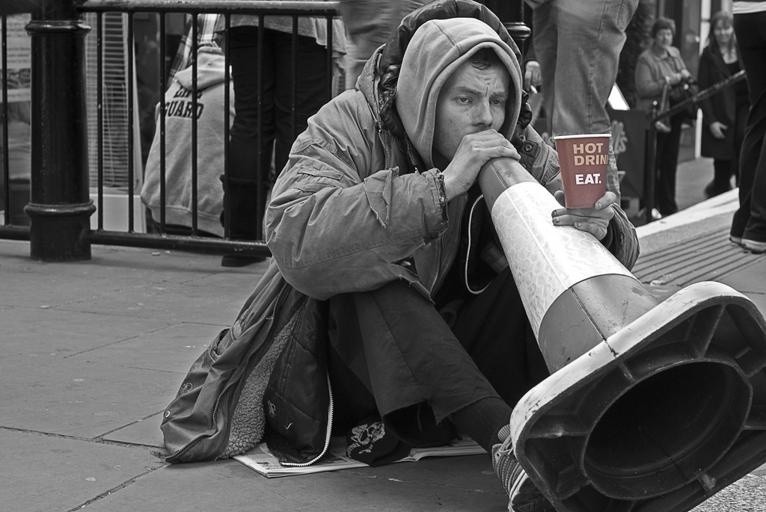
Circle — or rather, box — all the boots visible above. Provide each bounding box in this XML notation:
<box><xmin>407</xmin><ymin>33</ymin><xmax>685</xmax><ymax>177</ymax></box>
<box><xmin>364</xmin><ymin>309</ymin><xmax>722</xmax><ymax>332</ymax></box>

<box><xmin>219</xmin><ymin>174</ymin><xmax>260</xmax><ymax>267</ymax></box>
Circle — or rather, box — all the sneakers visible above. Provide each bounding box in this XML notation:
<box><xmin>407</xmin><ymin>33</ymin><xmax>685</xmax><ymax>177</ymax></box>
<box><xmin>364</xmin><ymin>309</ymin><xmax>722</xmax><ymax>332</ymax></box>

<box><xmin>730</xmin><ymin>208</ymin><xmax>766</xmax><ymax>252</ymax></box>
<box><xmin>492</xmin><ymin>424</ymin><xmax>557</xmax><ymax>512</ymax></box>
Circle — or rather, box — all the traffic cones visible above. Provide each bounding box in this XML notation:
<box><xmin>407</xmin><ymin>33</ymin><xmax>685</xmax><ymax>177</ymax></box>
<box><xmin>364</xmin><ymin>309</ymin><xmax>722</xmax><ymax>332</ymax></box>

<box><xmin>477</xmin><ymin>158</ymin><xmax>766</xmax><ymax>512</ymax></box>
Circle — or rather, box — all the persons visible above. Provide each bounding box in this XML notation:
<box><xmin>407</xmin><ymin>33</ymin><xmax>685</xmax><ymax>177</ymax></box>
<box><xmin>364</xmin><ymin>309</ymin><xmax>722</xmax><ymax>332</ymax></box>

<box><xmin>611</xmin><ymin>0</ymin><xmax>766</xmax><ymax>255</ymax></box>
<box><xmin>142</xmin><ymin>1</ymin><xmax>638</xmax><ymax>256</ymax></box>
<box><xmin>159</xmin><ymin>0</ymin><xmax>641</xmax><ymax>512</ymax></box>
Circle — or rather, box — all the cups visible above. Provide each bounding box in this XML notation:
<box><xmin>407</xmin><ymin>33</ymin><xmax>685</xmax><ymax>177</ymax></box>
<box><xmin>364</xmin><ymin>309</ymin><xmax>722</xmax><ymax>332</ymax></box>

<box><xmin>554</xmin><ymin>134</ymin><xmax>608</xmax><ymax>210</ymax></box>
